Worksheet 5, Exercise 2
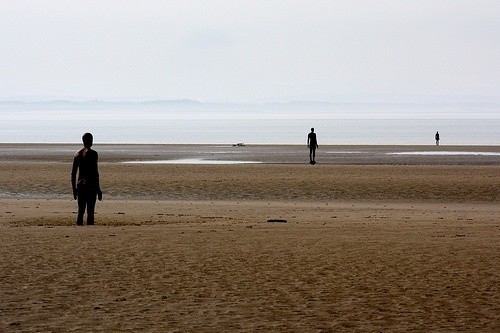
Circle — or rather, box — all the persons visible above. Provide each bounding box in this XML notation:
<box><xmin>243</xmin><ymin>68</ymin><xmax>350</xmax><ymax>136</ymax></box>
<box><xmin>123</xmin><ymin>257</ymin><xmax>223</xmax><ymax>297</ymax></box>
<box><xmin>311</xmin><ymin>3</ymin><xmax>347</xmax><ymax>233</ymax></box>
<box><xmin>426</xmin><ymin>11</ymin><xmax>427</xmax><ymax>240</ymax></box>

<box><xmin>307</xmin><ymin>128</ymin><xmax>319</xmax><ymax>164</ymax></box>
<box><xmin>69</xmin><ymin>133</ymin><xmax>102</xmax><ymax>226</ymax></box>
<box><xmin>435</xmin><ymin>131</ymin><xmax>440</xmax><ymax>145</ymax></box>
